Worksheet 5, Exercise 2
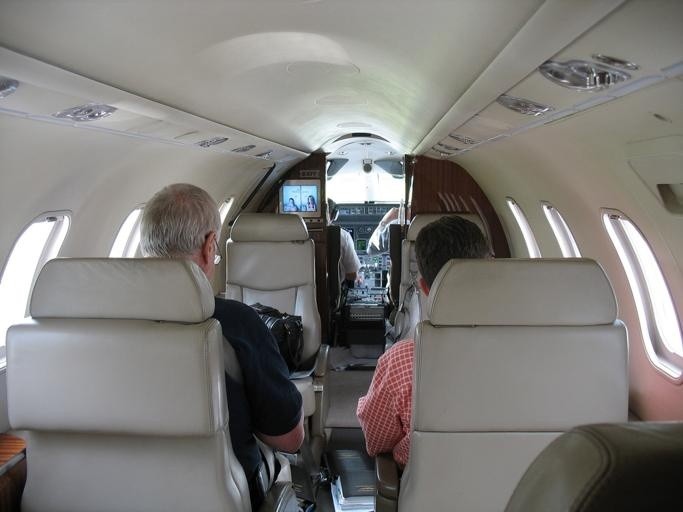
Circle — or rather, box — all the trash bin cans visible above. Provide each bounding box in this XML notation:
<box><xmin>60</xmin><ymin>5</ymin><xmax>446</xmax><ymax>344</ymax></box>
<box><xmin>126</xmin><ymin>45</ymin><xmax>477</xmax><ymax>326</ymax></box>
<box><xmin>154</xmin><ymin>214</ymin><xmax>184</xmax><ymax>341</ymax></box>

<box><xmin>380</xmin><ymin>220</ymin><xmax>386</xmax><ymax>226</ymax></box>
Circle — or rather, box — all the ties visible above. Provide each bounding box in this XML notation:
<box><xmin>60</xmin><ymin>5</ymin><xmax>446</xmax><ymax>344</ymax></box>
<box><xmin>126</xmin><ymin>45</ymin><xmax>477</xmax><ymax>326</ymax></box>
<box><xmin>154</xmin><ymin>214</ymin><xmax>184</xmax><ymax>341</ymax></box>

<box><xmin>278</xmin><ymin>179</ymin><xmax>321</xmax><ymax>218</ymax></box>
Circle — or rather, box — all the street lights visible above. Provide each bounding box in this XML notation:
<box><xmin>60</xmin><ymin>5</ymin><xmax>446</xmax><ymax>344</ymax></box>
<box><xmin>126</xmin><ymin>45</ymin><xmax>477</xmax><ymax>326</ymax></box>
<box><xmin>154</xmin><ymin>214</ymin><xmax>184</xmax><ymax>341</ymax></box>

<box><xmin>247</xmin><ymin>303</ymin><xmax>303</xmax><ymax>376</ymax></box>
<box><xmin>315</xmin><ymin>474</ymin><xmax>343</xmax><ymax>512</ymax></box>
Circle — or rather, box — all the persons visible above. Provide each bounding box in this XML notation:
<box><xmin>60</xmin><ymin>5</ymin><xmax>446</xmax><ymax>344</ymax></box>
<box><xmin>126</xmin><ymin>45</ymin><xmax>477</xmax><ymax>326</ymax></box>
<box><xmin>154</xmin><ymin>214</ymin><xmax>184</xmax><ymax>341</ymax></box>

<box><xmin>366</xmin><ymin>203</ymin><xmax>411</xmax><ymax>295</ymax></box>
<box><xmin>140</xmin><ymin>184</ymin><xmax>305</xmax><ymax>512</ymax></box>
<box><xmin>307</xmin><ymin>196</ymin><xmax>316</xmax><ymax>211</ymax></box>
<box><xmin>356</xmin><ymin>216</ymin><xmax>492</xmax><ymax>472</ymax></box>
<box><xmin>328</xmin><ymin>199</ymin><xmax>362</xmax><ymax>284</ymax></box>
<box><xmin>284</xmin><ymin>198</ymin><xmax>298</xmax><ymax>212</ymax></box>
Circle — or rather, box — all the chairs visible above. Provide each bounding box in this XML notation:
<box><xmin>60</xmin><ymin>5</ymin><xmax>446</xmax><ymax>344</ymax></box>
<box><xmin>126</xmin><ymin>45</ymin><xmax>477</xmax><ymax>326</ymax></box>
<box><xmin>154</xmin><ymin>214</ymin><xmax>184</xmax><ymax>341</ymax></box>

<box><xmin>295</xmin><ymin>449</ymin><xmax>303</xmax><ymax>468</ymax></box>
<box><xmin>374</xmin><ymin>213</ymin><xmax>683</xmax><ymax>512</ymax></box>
<box><xmin>5</xmin><ymin>212</ymin><xmax>352</xmax><ymax>512</ymax></box>
